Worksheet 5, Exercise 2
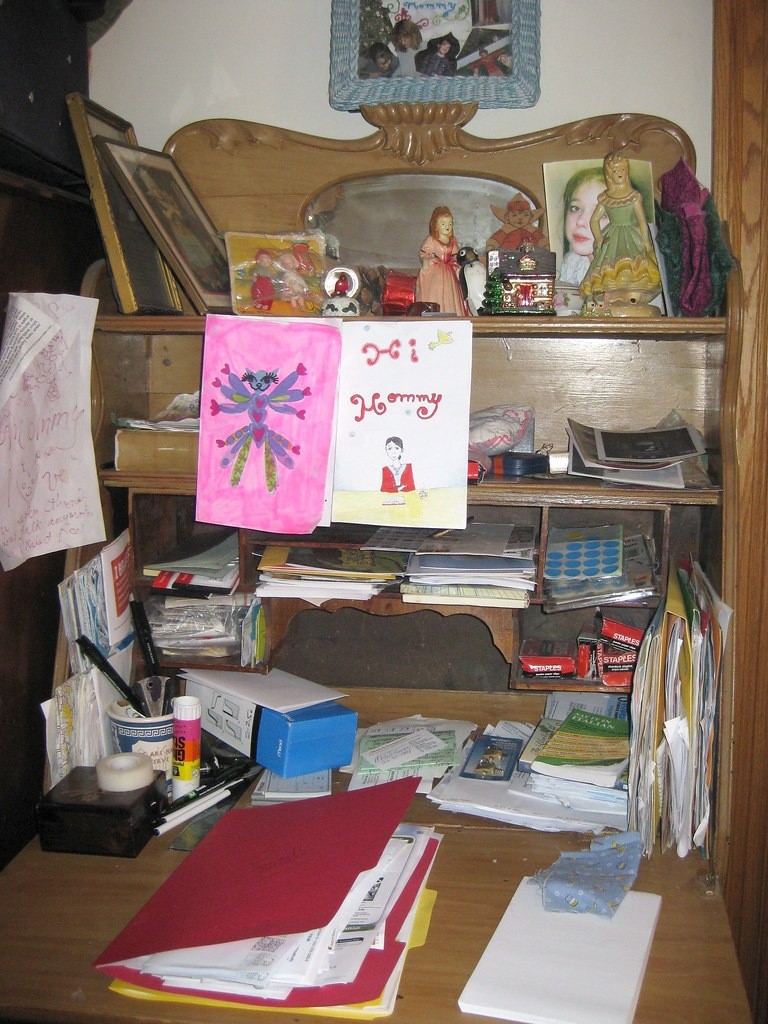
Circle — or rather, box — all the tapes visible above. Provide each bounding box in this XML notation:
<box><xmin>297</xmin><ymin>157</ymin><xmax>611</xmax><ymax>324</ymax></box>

<box><xmin>96</xmin><ymin>751</ymin><xmax>153</xmax><ymax>793</ymax></box>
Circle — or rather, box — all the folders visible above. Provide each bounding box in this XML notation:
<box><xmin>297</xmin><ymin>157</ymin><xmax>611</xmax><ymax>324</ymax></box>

<box><xmin>649</xmin><ymin>553</ymin><xmax>694</xmax><ymax>845</ymax></box>
<box><xmin>92</xmin><ymin>774</ymin><xmax>439</xmax><ymax>1008</ymax></box>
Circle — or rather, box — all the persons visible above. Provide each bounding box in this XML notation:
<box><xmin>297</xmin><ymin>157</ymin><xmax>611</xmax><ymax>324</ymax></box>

<box><xmin>580</xmin><ymin>151</ymin><xmax>664</xmax><ymax>303</ymax></box>
<box><xmin>558</xmin><ymin>169</ymin><xmax>636</xmax><ymax>288</ymax></box>
<box><xmin>415</xmin><ymin>206</ymin><xmax>468</xmax><ymax>317</ymax></box>
<box><xmin>250</xmin><ymin>250</ymin><xmax>310</xmax><ymax>312</ymax></box>
<box><xmin>357</xmin><ymin>17</ymin><xmax>513</xmax><ymax>80</ymax></box>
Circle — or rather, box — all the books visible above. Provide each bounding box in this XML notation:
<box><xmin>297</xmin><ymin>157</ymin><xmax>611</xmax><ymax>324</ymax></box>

<box><xmin>34</xmin><ymin>314</ymin><xmax>738</xmax><ymax>1024</ymax></box>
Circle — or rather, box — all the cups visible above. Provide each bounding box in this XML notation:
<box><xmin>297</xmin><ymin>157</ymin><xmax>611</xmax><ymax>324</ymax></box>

<box><xmin>105</xmin><ymin>699</ymin><xmax>172</xmax><ymax>799</ymax></box>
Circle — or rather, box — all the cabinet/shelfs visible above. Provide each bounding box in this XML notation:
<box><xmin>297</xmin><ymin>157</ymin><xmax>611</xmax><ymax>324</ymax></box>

<box><xmin>52</xmin><ymin>100</ymin><xmax>728</xmax><ymax>726</ymax></box>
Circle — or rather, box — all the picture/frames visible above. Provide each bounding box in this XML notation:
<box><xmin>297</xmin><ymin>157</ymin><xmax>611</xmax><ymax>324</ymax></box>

<box><xmin>93</xmin><ymin>135</ymin><xmax>233</xmax><ymax>314</ymax></box>
<box><xmin>64</xmin><ymin>91</ymin><xmax>183</xmax><ymax>314</ymax></box>
<box><xmin>327</xmin><ymin>0</ymin><xmax>541</xmax><ymax>111</ymax></box>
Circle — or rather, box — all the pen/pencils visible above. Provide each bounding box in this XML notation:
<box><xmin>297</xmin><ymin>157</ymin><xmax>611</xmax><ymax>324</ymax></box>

<box><xmin>127</xmin><ymin>592</ymin><xmax>175</xmax><ymax>715</ymax></box>
<box><xmin>74</xmin><ymin>634</ymin><xmax>145</xmax><ymax>715</ymax></box>
<box><xmin>152</xmin><ymin>761</ymin><xmax>252</xmax><ymax>837</ymax></box>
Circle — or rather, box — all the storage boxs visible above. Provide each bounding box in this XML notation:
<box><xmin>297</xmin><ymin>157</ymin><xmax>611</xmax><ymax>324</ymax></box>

<box><xmin>186</xmin><ymin>679</ymin><xmax>359</xmax><ymax>778</ymax></box>
<box><xmin>517</xmin><ymin>608</ymin><xmax>650</xmax><ymax>688</ymax></box>
<box><xmin>114</xmin><ymin>428</ymin><xmax>199</xmax><ymax>472</ymax></box>
<box><xmin>36</xmin><ymin>766</ymin><xmax>166</xmax><ymax>859</ymax></box>
